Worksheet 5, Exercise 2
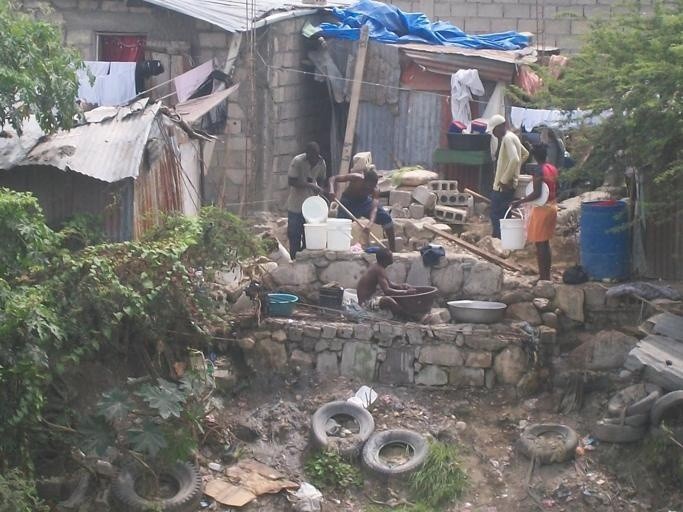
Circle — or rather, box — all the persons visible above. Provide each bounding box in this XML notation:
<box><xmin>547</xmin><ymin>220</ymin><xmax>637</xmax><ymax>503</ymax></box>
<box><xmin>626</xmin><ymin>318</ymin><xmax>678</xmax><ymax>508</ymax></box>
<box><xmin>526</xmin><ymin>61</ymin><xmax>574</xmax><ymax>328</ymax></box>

<box><xmin>286</xmin><ymin>140</ymin><xmax>329</xmax><ymax>260</ymax></box>
<box><xmin>329</xmin><ymin>169</ymin><xmax>396</xmax><ymax>251</ymax></box>
<box><xmin>508</xmin><ymin>143</ymin><xmax>559</xmax><ymax>280</ymax></box>
<box><xmin>356</xmin><ymin>248</ymin><xmax>433</xmax><ymax>325</ymax></box>
<box><xmin>485</xmin><ymin>114</ymin><xmax>529</xmax><ymax>238</ymax></box>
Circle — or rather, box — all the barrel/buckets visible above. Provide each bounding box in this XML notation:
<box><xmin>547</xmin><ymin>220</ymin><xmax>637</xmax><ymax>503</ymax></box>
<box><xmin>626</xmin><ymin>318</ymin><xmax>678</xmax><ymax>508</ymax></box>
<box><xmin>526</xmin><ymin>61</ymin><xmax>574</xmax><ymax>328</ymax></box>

<box><xmin>301</xmin><ymin>194</ymin><xmax>328</xmax><ymax>222</ymax></box>
<box><xmin>500</xmin><ymin>205</ymin><xmax>526</xmax><ymax>251</ymax></box>
<box><xmin>581</xmin><ymin>201</ymin><xmax>632</xmax><ymax>277</ymax></box>
<box><xmin>303</xmin><ymin>223</ymin><xmax>328</xmax><ymax>249</ymax></box>
<box><xmin>328</xmin><ymin>216</ymin><xmax>352</xmax><ymax>251</ymax></box>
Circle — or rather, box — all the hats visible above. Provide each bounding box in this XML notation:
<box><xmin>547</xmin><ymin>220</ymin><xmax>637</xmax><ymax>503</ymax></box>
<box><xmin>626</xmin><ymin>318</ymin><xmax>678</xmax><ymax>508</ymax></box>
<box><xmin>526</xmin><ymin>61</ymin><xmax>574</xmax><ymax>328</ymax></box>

<box><xmin>484</xmin><ymin>114</ymin><xmax>505</xmax><ymax>133</ymax></box>
